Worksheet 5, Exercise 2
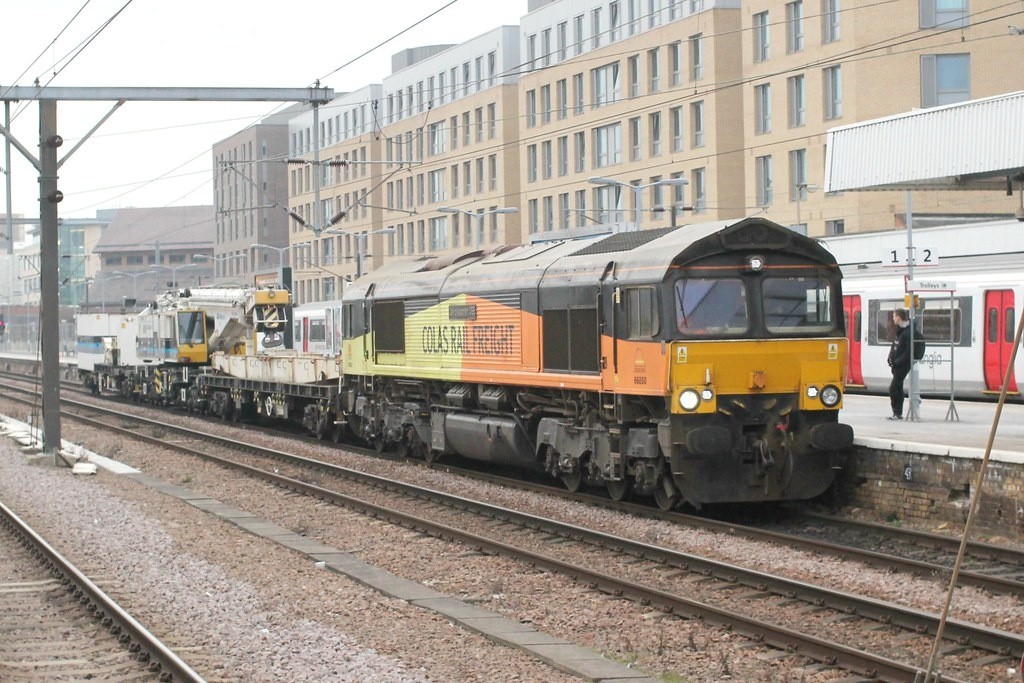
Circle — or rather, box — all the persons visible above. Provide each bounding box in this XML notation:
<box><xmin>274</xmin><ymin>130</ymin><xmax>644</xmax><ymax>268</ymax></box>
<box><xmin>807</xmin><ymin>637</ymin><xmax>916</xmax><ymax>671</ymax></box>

<box><xmin>887</xmin><ymin>308</ymin><xmax>916</xmax><ymax>419</ymax></box>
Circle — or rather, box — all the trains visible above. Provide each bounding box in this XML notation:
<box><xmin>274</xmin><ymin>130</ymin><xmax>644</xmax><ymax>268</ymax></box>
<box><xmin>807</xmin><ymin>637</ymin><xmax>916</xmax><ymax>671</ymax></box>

<box><xmin>74</xmin><ymin>215</ymin><xmax>854</xmax><ymax>519</ymax></box>
<box><xmin>293</xmin><ymin>251</ymin><xmax>1024</xmax><ymax>407</ymax></box>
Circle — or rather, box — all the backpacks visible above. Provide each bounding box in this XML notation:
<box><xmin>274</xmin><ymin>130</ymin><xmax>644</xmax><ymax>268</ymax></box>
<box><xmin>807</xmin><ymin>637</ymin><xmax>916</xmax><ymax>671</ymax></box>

<box><xmin>910</xmin><ymin>326</ymin><xmax>925</xmax><ymax>359</ymax></box>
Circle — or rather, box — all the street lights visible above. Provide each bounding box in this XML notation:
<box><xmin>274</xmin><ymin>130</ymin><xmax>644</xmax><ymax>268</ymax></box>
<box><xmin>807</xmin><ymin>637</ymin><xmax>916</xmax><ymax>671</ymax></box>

<box><xmin>250</xmin><ymin>242</ymin><xmax>312</xmax><ymax>290</ymax></box>
<box><xmin>86</xmin><ymin>274</ymin><xmax>122</xmax><ymax>313</ymax></box>
<box><xmin>150</xmin><ymin>263</ymin><xmax>197</xmax><ymax>290</ymax></box>
<box><xmin>434</xmin><ymin>206</ymin><xmax>519</xmax><ymax>250</ymax></box>
<box><xmin>586</xmin><ymin>174</ymin><xmax>690</xmax><ymax>232</ymax></box>
<box><xmin>323</xmin><ymin>228</ymin><xmax>396</xmax><ymax>277</ymax></box>
<box><xmin>112</xmin><ymin>269</ymin><xmax>156</xmax><ymax>313</ymax></box>
<box><xmin>193</xmin><ymin>253</ymin><xmax>248</xmax><ymax>284</ymax></box>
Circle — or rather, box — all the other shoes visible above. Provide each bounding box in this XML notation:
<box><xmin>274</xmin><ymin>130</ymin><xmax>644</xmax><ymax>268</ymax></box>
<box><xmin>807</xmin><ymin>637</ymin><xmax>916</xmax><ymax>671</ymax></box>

<box><xmin>888</xmin><ymin>413</ymin><xmax>903</xmax><ymax>421</ymax></box>
